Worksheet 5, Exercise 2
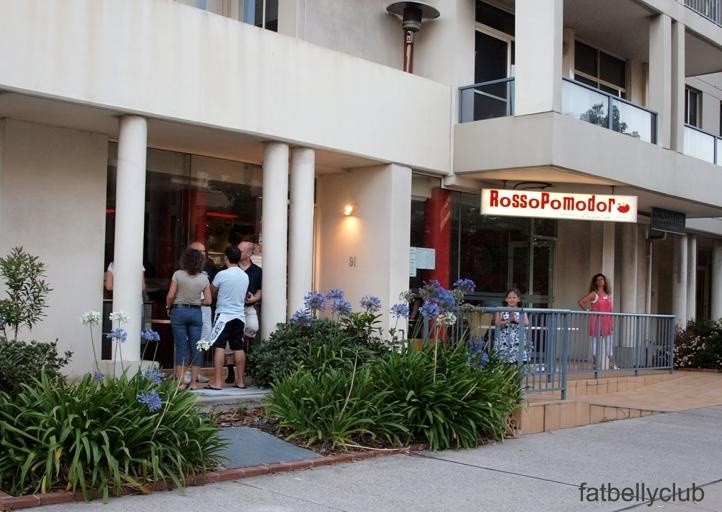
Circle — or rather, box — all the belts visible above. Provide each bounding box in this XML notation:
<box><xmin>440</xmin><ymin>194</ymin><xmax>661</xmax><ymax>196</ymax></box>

<box><xmin>170</xmin><ymin>304</ymin><xmax>201</xmax><ymax>309</ymax></box>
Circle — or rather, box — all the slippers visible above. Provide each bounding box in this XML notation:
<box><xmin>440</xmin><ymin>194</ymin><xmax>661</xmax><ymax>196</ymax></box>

<box><xmin>204</xmin><ymin>384</ymin><xmax>221</xmax><ymax>390</ymax></box>
<box><xmin>232</xmin><ymin>384</ymin><xmax>246</xmax><ymax>389</ymax></box>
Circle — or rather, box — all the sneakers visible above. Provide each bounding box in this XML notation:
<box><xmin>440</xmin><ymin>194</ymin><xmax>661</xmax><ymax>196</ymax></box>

<box><xmin>243</xmin><ymin>335</ymin><xmax>253</xmax><ymax>353</ymax></box>
<box><xmin>225</xmin><ymin>371</ymin><xmax>235</xmax><ymax>383</ymax></box>
<box><xmin>196</xmin><ymin>374</ymin><xmax>209</xmax><ymax>382</ymax></box>
<box><xmin>184</xmin><ymin>370</ymin><xmax>191</xmax><ymax>384</ymax></box>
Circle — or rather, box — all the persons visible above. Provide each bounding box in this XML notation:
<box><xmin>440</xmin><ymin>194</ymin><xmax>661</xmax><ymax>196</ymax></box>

<box><xmin>104</xmin><ymin>250</ymin><xmax>149</xmax><ymax>327</ymax></box>
<box><xmin>493</xmin><ymin>288</ymin><xmax>530</xmax><ymax>388</ymax></box>
<box><xmin>577</xmin><ymin>273</ymin><xmax>620</xmax><ymax>371</ymax></box>
<box><xmin>165</xmin><ymin>247</ymin><xmax>212</xmax><ymax>389</ymax></box>
<box><xmin>226</xmin><ymin>242</ymin><xmax>262</xmax><ymax>384</ymax></box>
<box><xmin>180</xmin><ymin>242</ymin><xmax>217</xmax><ymax>383</ymax></box>
<box><xmin>204</xmin><ymin>245</ymin><xmax>249</xmax><ymax>390</ymax></box>
